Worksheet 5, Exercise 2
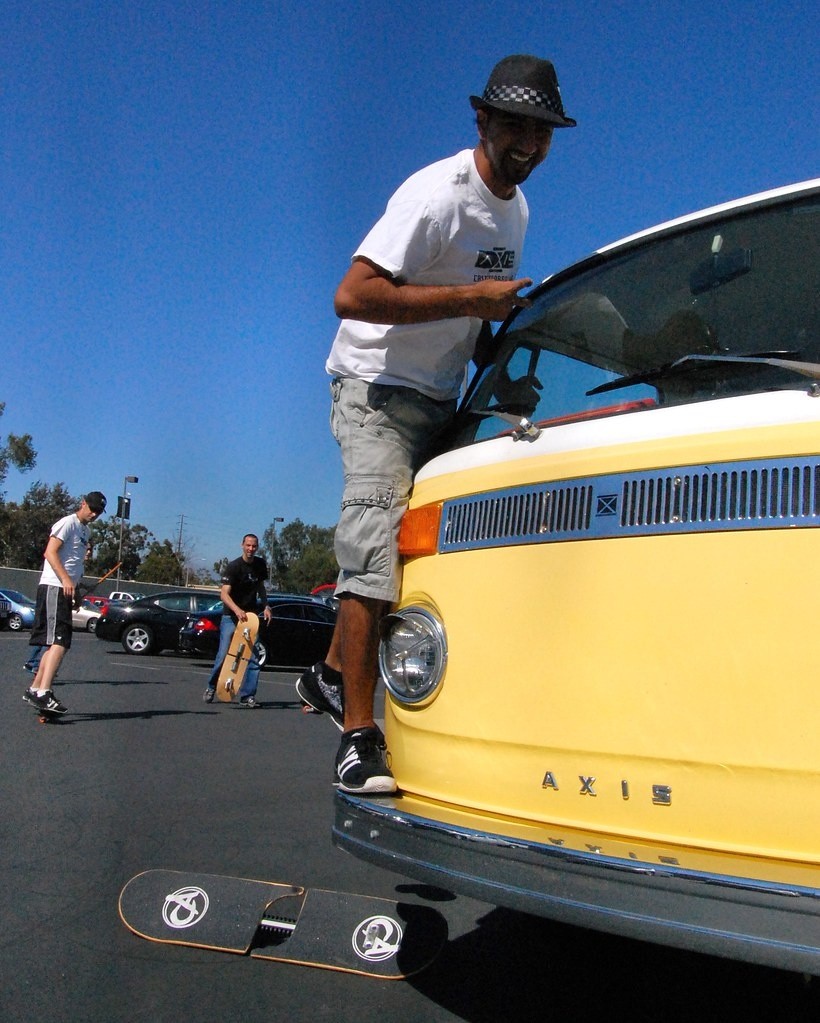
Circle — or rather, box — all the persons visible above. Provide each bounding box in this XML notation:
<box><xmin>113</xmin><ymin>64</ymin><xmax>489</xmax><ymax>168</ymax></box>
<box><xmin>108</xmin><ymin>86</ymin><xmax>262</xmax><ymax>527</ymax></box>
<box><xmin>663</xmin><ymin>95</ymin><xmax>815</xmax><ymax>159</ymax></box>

<box><xmin>22</xmin><ymin>491</ymin><xmax>107</xmax><ymax>713</ymax></box>
<box><xmin>22</xmin><ymin>537</ymin><xmax>93</xmax><ymax>676</ymax></box>
<box><xmin>203</xmin><ymin>534</ymin><xmax>272</xmax><ymax>707</ymax></box>
<box><xmin>295</xmin><ymin>54</ymin><xmax>578</xmax><ymax>793</ymax></box>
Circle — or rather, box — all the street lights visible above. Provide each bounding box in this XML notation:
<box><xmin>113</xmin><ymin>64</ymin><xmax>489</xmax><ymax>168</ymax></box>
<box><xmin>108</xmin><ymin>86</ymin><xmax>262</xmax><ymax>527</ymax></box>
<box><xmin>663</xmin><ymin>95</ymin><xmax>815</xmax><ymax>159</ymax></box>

<box><xmin>269</xmin><ymin>517</ymin><xmax>284</xmax><ymax>589</ymax></box>
<box><xmin>116</xmin><ymin>474</ymin><xmax>138</xmax><ymax>590</ymax></box>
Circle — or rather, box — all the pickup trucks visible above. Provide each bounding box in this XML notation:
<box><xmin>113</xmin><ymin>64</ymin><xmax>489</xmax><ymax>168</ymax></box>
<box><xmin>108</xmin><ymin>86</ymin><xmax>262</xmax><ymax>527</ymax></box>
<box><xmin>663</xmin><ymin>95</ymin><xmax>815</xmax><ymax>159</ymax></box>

<box><xmin>99</xmin><ymin>591</ymin><xmax>146</xmax><ymax>602</ymax></box>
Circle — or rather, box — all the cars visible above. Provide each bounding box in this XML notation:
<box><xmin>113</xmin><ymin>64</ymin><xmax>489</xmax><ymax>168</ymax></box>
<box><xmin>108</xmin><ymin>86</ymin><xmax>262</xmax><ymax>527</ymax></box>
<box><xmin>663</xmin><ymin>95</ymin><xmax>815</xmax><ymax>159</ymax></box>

<box><xmin>0</xmin><ymin>589</ymin><xmax>37</xmax><ymax>631</ymax></box>
<box><xmin>94</xmin><ymin>590</ymin><xmax>224</xmax><ymax>656</ymax></box>
<box><xmin>179</xmin><ymin>594</ymin><xmax>339</xmax><ymax>671</ymax></box>
<box><xmin>72</xmin><ymin>600</ymin><xmax>104</xmax><ymax>632</ymax></box>
<box><xmin>84</xmin><ymin>596</ymin><xmax>113</xmax><ymax>616</ymax></box>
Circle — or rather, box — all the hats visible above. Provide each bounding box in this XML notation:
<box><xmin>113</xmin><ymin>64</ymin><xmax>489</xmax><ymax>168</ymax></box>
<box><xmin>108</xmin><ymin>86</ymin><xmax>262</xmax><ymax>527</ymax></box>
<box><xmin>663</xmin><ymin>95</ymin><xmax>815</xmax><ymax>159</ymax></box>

<box><xmin>468</xmin><ymin>54</ymin><xmax>578</xmax><ymax>128</ymax></box>
<box><xmin>87</xmin><ymin>492</ymin><xmax>108</xmax><ymax>514</ymax></box>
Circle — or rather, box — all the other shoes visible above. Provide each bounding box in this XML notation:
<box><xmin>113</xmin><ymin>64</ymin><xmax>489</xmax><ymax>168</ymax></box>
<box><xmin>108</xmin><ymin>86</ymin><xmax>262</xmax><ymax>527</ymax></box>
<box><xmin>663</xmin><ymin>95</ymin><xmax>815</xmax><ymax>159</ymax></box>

<box><xmin>22</xmin><ymin>663</ymin><xmax>34</xmax><ymax>671</ymax></box>
<box><xmin>32</xmin><ymin>666</ymin><xmax>59</xmax><ymax>676</ymax></box>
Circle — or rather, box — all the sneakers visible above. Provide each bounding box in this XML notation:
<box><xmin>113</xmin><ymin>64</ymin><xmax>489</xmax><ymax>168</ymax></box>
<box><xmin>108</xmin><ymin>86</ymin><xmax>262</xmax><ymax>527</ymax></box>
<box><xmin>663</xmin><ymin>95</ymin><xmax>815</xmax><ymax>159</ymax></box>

<box><xmin>240</xmin><ymin>697</ymin><xmax>260</xmax><ymax>708</ymax></box>
<box><xmin>22</xmin><ymin>688</ymin><xmax>35</xmax><ymax>701</ymax></box>
<box><xmin>331</xmin><ymin>727</ymin><xmax>397</xmax><ymax>794</ymax></box>
<box><xmin>295</xmin><ymin>662</ymin><xmax>345</xmax><ymax>734</ymax></box>
<box><xmin>202</xmin><ymin>688</ymin><xmax>214</xmax><ymax>703</ymax></box>
<box><xmin>29</xmin><ymin>690</ymin><xmax>69</xmax><ymax>713</ymax></box>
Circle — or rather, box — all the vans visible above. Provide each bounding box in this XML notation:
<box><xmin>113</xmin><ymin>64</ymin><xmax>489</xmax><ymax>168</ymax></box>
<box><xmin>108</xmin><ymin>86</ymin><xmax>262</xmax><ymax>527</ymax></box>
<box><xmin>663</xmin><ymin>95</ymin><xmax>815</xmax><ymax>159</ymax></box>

<box><xmin>327</xmin><ymin>176</ymin><xmax>819</xmax><ymax>984</ymax></box>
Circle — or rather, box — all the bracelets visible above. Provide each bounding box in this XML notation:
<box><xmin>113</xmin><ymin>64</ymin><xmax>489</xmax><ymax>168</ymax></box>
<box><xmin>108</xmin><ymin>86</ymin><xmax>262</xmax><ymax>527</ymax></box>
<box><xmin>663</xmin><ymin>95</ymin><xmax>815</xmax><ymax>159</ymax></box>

<box><xmin>265</xmin><ymin>605</ymin><xmax>271</xmax><ymax>611</ymax></box>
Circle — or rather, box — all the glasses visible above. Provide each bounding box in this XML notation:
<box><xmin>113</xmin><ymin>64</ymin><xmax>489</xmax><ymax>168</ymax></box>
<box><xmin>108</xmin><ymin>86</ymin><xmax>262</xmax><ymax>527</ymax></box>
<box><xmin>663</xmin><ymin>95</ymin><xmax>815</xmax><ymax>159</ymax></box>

<box><xmin>87</xmin><ymin>504</ymin><xmax>104</xmax><ymax>514</ymax></box>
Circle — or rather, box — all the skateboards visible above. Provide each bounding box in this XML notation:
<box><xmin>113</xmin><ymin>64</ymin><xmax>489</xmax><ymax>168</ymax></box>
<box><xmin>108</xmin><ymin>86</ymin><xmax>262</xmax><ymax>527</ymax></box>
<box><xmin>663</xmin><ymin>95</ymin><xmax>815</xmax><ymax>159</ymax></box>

<box><xmin>117</xmin><ymin>867</ymin><xmax>448</xmax><ymax>981</ymax></box>
<box><xmin>26</xmin><ymin>664</ymin><xmax>61</xmax><ymax>679</ymax></box>
<box><xmin>215</xmin><ymin>610</ymin><xmax>260</xmax><ymax>703</ymax></box>
<box><xmin>27</xmin><ymin>703</ymin><xmax>63</xmax><ymax>724</ymax></box>
